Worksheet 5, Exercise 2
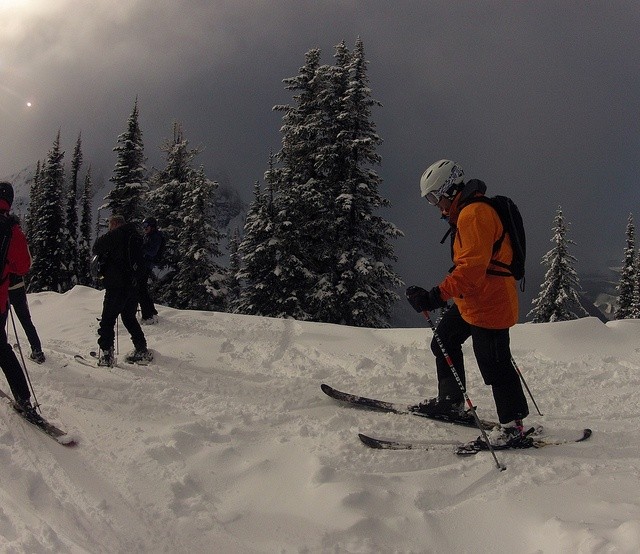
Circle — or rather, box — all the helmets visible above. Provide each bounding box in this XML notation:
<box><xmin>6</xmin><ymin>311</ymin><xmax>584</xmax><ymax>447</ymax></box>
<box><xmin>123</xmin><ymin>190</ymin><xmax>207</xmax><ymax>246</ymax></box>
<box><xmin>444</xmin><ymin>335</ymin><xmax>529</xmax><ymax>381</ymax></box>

<box><xmin>140</xmin><ymin>216</ymin><xmax>158</xmax><ymax>227</ymax></box>
<box><xmin>1</xmin><ymin>182</ymin><xmax>14</xmax><ymax>207</ymax></box>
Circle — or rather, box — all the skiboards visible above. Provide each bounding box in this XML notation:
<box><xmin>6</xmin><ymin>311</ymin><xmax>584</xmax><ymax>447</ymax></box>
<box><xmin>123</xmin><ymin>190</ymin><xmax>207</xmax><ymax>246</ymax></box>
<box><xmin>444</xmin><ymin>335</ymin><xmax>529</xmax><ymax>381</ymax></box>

<box><xmin>74</xmin><ymin>352</ymin><xmax>153</xmax><ymax>367</ymax></box>
<box><xmin>0</xmin><ymin>389</ymin><xmax>76</xmax><ymax>447</ymax></box>
<box><xmin>320</xmin><ymin>384</ymin><xmax>592</xmax><ymax>450</ymax></box>
<box><xmin>13</xmin><ymin>344</ymin><xmax>68</xmax><ymax>368</ymax></box>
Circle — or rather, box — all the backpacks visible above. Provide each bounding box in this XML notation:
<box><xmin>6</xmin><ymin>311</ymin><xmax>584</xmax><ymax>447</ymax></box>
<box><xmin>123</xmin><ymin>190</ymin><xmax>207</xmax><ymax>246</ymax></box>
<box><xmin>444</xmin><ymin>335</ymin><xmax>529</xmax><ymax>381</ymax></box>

<box><xmin>438</xmin><ymin>194</ymin><xmax>527</xmax><ymax>292</ymax></box>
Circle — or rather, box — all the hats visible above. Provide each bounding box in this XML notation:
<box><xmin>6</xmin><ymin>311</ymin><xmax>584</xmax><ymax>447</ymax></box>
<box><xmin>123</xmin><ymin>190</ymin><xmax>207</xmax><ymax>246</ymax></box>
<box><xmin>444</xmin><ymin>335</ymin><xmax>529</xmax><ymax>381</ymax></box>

<box><xmin>418</xmin><ymin>157</ymin><xmax>466</xmax><ymax>199</ymax></box>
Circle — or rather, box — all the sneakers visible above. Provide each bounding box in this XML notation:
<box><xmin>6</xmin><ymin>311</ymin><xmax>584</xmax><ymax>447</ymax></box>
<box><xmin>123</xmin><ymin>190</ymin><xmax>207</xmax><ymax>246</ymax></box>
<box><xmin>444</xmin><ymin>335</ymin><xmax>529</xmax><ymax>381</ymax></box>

<box><xmin>417</xmin><ymin>394</ymin><xmax>466</xmax><ymax>417</ymax></box>
<box><xmin>476</xmin><ymin>419</ymin><xmax>524</xmax><ymax>447</ymax></box>
<box><xmin>99</xmin><ymin>353</ymin><xmax>114</xmax><ymax>367</ymax></box>
<box><xmin>12</xmin><ymin>400</ymin><xmax>33</xmax><ymax>414</ymax></box>
<box><xmin>30</xmin><ymin>350</ymin><xmax>46</xmax><ymax>362</ymax></box>
<box><xmin>127</xmin><ymin>348</ymin><xmax>147</xmax><ymax>360</ymax></box>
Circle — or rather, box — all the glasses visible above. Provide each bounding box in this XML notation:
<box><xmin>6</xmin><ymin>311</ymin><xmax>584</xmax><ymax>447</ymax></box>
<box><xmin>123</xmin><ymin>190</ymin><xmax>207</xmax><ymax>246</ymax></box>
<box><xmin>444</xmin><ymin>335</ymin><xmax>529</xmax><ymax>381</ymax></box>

<box><xmin>423</xmin><ymin>164</ymin><xmax>461</xmax><ymax>206</ymax></box>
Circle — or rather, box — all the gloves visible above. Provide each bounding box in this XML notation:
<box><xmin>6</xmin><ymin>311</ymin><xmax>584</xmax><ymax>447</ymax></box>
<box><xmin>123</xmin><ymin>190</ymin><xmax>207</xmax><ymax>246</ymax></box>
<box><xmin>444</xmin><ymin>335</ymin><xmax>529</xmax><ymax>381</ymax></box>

<box><xmin>404</xmin><ymin>286</ymin><xmax>447</xmax><ymax>313</ymax></box>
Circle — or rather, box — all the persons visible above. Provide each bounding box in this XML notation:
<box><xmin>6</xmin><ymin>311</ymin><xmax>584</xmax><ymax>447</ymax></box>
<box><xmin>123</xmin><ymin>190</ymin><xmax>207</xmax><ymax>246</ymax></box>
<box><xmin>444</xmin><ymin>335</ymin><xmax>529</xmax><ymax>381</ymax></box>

<box><xmin>92</xmin><ymin>215</ymin><xmax>153</xmax><ymax>367</ymax></box>
<box><xmin>0</xmin><ymin>181</ymin><xmax>33</xmax><ymax>413</ymax></box>
<box><xmin>5</xmin><ymin>214</ymin><xmax>46</xmax><ymax>362</ymax></box>
<box><xmin>137</xmin><ymin>216</ymin><xmax>161</xmax><ymax>325</ymax></box>
<box><xmin>405</xmin><ymin>157</ymin><xmax>533</xmax><ymax>449</ymax></box>
